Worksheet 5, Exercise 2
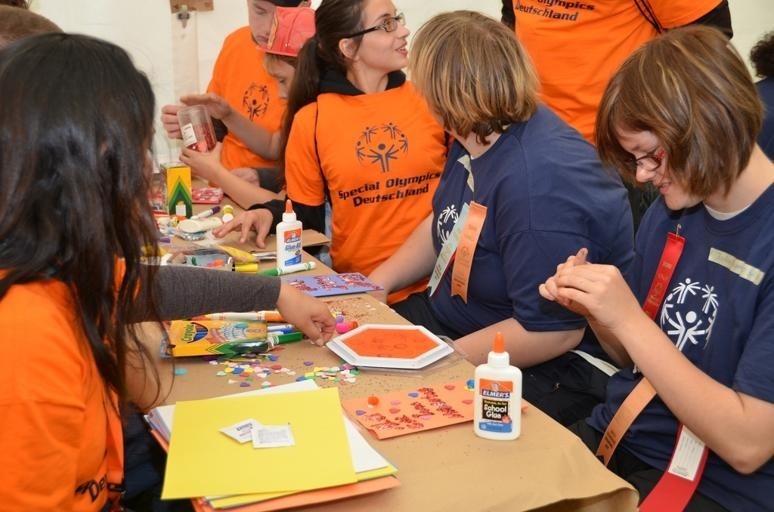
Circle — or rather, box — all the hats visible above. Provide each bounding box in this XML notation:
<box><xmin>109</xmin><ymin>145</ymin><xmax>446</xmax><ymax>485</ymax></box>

<box><xmin>256</xmin><ymin>7</ymin><xmax>317</xmax><ymax>57</ymax></box>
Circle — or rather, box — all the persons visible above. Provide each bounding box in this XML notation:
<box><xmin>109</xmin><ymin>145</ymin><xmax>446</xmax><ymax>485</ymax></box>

<box><xmin>0</xmin><ymin>5</ymin><xmax>64</xmax><ymax>48</ymax></box>
<box><xmin>0</xmin><ymin>33</ymin><xmax>336</xmax><ymax>512</ymax></box>
<box><xmin>367</xmin><ymin>11</ymin><xmax>635</xmax><ymax>428</ymax></box>
<box><xmin>502</xmin><ymin>0</ymin><xmax>733</xmax><ymax>240</ymax></box>
<box><xmin>212</xmin><ymin>0</ymin><xmax>456</xmax><ymax>306</ymax></box>
<box><xmin>538</xmin><ymin>25</ymin><xmax>774</xmax><ymax>512</ymax></box>
<box><xmin>179</xmin><ymin>53</ymin><xmax>332</xmax><ymax>270</ymax></box>
<box><xmin>750</xmin><ymin>31</ymin><xmax>774</xmax><ymax>162</ymax></box>
<box><xmin>0</xmin><ymin>0</ymin><xmax>29</xmax><ymax>9</ymax></box>
<box><xmin>161</xmin><ymin>0</ymin><xmax>312</xmax><ymax>188</ymax></box>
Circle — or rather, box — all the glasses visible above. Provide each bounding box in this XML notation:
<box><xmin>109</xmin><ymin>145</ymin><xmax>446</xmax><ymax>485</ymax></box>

<box><xmin>623</xmin><ymin>145</ymin><xmax>668</xmax><ymax>174</ymax></box>
<box><xmin>335</xmin><ymin>12</ymin><xmax>406</xmax><ymax>51</ymax></box>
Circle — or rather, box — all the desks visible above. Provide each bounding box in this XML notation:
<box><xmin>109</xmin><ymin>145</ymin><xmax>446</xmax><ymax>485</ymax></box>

<box><xmin>121</xmin><ymin>175</ymin><xmax>642</xmax><ymax>512</ymax></box>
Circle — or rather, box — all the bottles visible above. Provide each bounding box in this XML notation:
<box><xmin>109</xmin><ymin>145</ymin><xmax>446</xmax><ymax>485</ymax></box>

<box><xmin>473</xmin><ymin>331</ymin><xmax>523</xmax><ymax>440</ymax></box>
<box><xmin>177</xmin><ymin>104</ymin><xmax>224</xmax><ymax>188</ymax></box>
<box><xmin>275</xmin><ymin>199</ymin><xmax>303</xmax><ymax>269</ymax></box>
